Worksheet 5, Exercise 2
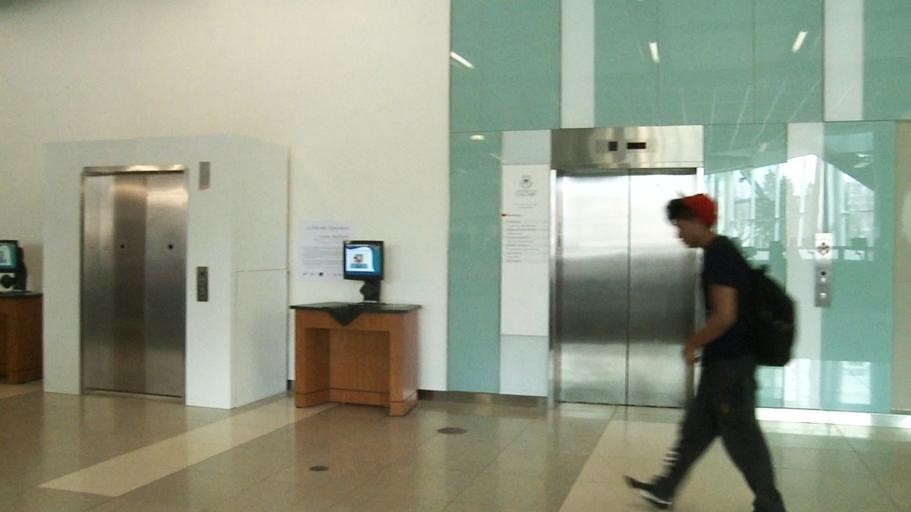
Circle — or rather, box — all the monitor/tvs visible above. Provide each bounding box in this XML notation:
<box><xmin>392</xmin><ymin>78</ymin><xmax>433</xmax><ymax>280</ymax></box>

<box><xmin>0</xmin><ymin>239</ymin><xmax>24</xmax><ymax>271</ymax></box>
<box><xmin>343</xmin><ymin>239</ymin><xmax>384</xmax><ymax>279</ymax></box>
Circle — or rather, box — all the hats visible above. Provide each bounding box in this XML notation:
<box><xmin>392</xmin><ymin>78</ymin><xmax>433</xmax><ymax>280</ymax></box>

<box><xmin>681</xmin><ymin>192</ymin><xmax>717</xmax><ymax>229</ymax></box>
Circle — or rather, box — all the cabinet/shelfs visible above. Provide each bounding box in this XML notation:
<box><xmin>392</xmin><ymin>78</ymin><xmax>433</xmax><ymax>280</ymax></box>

<box><xmin>0</xmin><ymin>291</ymin><xmax>43</xmax><ymax>385</ymax></box>
<box><xmin>288</xmin><ymin>302</ymin><xmax>421</xmax><ymax>417</ymax></box>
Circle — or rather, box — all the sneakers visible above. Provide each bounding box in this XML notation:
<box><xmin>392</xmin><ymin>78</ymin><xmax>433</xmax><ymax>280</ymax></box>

<box><xmin>623</xmin><ymin>475</ymin><xmax>674</xmax><ymax>509</ymax></box>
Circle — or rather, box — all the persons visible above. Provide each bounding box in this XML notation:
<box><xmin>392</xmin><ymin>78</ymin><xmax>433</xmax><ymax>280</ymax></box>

<box><xmin>621</xmin><ymin>189</ymin><xmax>786</xmax><ymax>511</ymax></box>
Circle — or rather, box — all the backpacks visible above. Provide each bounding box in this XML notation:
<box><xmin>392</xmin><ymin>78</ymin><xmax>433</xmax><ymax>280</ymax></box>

<box><xmin>717</xmin><ymin>235</ymin><xmax>795</xmax><ymax>367</ymax></box>
